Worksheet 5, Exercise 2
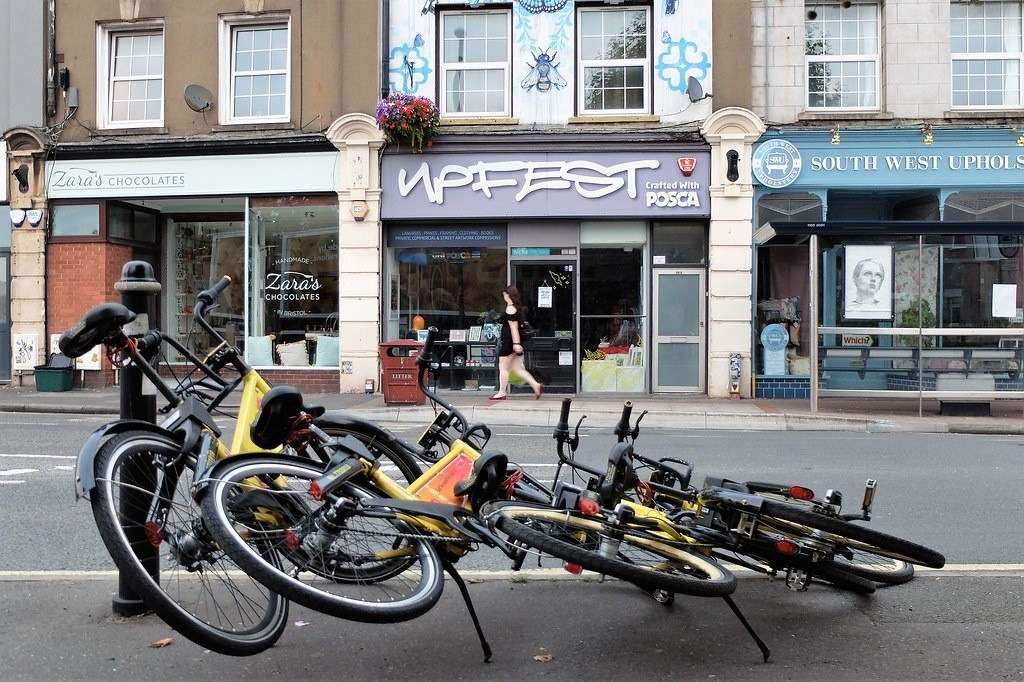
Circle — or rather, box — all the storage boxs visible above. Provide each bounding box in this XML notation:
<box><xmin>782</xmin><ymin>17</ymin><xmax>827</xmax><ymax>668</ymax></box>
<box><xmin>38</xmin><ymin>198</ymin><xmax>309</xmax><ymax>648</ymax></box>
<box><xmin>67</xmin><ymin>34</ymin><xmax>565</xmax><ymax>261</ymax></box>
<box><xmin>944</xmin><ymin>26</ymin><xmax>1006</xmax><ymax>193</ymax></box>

<box><xmin>433</xmin><ymin>346</ymin><xmax>499</xmax><ymax>391</ymax></box>
<box><xmin>581</xmin><ymin>342</ymin><xmax>647</xmax><ymax>392</ymax></box>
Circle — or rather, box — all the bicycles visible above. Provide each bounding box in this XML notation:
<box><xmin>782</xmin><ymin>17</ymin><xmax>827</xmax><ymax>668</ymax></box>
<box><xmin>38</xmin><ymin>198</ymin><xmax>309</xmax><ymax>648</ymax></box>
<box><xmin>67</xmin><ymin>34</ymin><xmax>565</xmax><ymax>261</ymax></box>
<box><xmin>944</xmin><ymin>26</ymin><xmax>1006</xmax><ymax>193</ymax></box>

<box><xmin>57</xmin><ymin>277</ymin><xmax>427</xmax><ymax>658</ymax></box>
<box><xmin>191</xmin><ymin>325</ymin><xmax>945</xmax><ymax>665</ymax></box>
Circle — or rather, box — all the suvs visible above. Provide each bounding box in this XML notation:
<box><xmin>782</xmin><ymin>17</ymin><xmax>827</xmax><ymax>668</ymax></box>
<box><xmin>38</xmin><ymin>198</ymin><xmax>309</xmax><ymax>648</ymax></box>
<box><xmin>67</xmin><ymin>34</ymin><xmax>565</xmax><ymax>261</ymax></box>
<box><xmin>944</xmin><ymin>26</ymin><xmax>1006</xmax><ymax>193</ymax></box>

<box><xmin>404</xmin><ymin>321</ymin><xmax>467</xmax><ymax>366</ymax></box>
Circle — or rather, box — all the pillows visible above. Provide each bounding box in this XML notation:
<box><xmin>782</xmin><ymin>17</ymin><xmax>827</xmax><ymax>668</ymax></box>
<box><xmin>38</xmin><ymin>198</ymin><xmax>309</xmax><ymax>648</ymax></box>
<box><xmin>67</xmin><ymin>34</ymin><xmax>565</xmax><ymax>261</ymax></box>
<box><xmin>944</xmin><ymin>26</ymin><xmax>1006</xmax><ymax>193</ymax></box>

<box><xmin>276</xmin><ymin>339</ymin><xmax>310</xmax><ymax>365</ymax></box>
<box><xmin>754</xmin><ymin>320</ymin><xmax>802</xmax><ymax>346</ymax></box>
<box><xmin>244</xmin><ymin>334</ymin><xmax>276</xmax><ymax>366</ymax></box>
<box><xmin>759</xmin><ymin>293</ymin><xmax>801</xmax><ymax>321</ymax></box>
<box><xmin>316</xmin><ymin>337</ymin><xmax>338</xmax><ymax>366</ymax></box>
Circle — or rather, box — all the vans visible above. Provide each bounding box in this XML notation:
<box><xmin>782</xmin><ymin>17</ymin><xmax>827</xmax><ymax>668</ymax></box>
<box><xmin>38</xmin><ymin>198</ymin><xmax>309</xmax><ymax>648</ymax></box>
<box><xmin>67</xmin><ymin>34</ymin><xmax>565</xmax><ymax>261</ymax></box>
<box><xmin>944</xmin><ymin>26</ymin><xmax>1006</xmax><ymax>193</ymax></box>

<box><xmin>998</xmin><ymin>321</ymin><xmax>1024</xmax><ymax>350</ymax></box>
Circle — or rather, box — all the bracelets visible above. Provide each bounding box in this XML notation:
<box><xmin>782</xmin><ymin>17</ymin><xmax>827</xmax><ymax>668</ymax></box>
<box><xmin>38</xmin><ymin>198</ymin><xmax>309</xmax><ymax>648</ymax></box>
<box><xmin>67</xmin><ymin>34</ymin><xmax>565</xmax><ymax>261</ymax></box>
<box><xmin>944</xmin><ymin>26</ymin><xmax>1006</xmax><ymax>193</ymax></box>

<box><xmin>512</xmin><ymin>342</ymin><xmax>521</xmax><ymax>345</ymax></box>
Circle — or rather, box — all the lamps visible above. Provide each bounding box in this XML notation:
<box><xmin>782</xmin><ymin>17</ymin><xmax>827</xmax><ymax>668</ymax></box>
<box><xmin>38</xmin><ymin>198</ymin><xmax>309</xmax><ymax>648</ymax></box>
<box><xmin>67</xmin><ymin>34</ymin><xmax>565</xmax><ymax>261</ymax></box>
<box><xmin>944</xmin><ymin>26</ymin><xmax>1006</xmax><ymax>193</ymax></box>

<box><xmin>830</xmin><ymin>123</ymin><xmax>842</xmax><ymax>145</ymax></box>
<box><xmin>1016</xmin><ymin>136</ymin><xmax>1024</xmax><ymax>147</ymax></box>
<box><xmin>921</xmin><ymin>123</ymin><xmax>934</xmax><ymax>146</ymax></box>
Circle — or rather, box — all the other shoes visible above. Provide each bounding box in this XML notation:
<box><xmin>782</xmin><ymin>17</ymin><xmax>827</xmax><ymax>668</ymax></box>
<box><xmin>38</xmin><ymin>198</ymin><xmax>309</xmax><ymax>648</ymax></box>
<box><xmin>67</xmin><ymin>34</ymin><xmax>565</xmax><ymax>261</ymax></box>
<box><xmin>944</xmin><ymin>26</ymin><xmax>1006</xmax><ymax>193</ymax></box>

<box><xmin>536</xmin><ymin>384</ymin><xmax>542</xmax><ymax>399</ymax></box>
<box><xmin>489</xmin><ymin>396</ymin><xmax>506</xmax><ymax>400</ymax></box>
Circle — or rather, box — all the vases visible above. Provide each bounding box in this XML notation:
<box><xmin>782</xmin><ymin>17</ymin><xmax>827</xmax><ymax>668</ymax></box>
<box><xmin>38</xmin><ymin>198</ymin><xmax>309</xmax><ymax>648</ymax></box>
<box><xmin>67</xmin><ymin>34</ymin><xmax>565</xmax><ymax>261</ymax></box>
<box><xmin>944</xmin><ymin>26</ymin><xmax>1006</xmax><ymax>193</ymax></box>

<box><xmin>387</xmin><ymin>120</ymin><xmax>432</xmax><ymax>147</ymax></box>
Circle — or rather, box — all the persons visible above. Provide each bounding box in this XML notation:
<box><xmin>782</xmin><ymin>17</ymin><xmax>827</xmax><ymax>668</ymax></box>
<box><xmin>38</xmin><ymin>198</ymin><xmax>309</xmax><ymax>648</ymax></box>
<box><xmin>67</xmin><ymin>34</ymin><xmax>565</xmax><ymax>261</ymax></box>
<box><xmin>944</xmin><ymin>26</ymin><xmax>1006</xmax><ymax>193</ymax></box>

<box><xmin>488</xmin><ymin>285</ymin><xmax>543</xmax><ymax>400</ymax></box>
<box><xmin>406</xmin><ymin>316</ymin><xmax>425</xmax><ymax>340</ymax></box>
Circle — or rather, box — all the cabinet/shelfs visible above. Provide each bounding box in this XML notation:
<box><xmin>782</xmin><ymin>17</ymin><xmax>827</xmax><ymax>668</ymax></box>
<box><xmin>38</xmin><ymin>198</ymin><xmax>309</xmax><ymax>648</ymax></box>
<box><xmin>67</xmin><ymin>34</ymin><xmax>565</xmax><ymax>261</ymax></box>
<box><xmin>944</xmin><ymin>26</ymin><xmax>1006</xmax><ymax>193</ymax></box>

<box><xmin>176</xmin><ymin>228</ymin><xmax>211</xmax><ymax>358</ymax></box>
<box><xmin>431</xmin><ymin>340</ymin><xmax>501</xmax><ymax>373</ymax></box>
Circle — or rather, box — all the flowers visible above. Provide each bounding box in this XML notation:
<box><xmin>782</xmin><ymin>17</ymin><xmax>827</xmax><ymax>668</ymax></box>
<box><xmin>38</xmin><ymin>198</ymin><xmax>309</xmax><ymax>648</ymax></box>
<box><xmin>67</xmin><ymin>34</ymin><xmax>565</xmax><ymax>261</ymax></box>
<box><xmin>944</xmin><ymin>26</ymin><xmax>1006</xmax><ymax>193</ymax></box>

<box><xmin>376</xmin><ymin>89</ymin><xmax>441</xmax><ymax>151</ymax></box>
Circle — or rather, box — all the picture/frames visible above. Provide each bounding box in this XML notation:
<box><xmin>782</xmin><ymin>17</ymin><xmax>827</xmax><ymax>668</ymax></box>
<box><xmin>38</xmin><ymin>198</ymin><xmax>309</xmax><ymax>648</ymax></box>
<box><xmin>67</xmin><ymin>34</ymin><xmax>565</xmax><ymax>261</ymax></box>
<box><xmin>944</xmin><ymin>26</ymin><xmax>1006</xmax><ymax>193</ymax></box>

<box><xmin>842</xmin><ymin>244</ymin><xmax>897</xmax><ymax>322</ymax></box>
<box><xmin>417</xmin><ymin>329</ymin><xmax>431</xmax><ymax>342</ymax></box>
<box><xmin>449</xmin><ymin>330</ymin><xmax>466</xmax><ymax>342</ymax></box>
<box><xmin>469</xmin><ymin>326</ymin><xmax>483</xmax><ymax>342</ymax></box>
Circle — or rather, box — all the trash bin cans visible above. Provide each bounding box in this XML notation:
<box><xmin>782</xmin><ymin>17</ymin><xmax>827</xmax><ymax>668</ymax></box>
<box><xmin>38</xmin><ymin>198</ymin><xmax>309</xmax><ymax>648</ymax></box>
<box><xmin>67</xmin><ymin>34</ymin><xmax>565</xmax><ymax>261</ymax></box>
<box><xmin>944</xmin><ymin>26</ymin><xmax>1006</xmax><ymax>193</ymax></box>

<box><xmin>34</xmin><ymin>353</ymin><xmax>76</xmax><ymax>392</ymax></box>
<box><xmin>379</xmin><ymin>338</ymin><xmax>428</xmax><ymax>406</ymax></box>
